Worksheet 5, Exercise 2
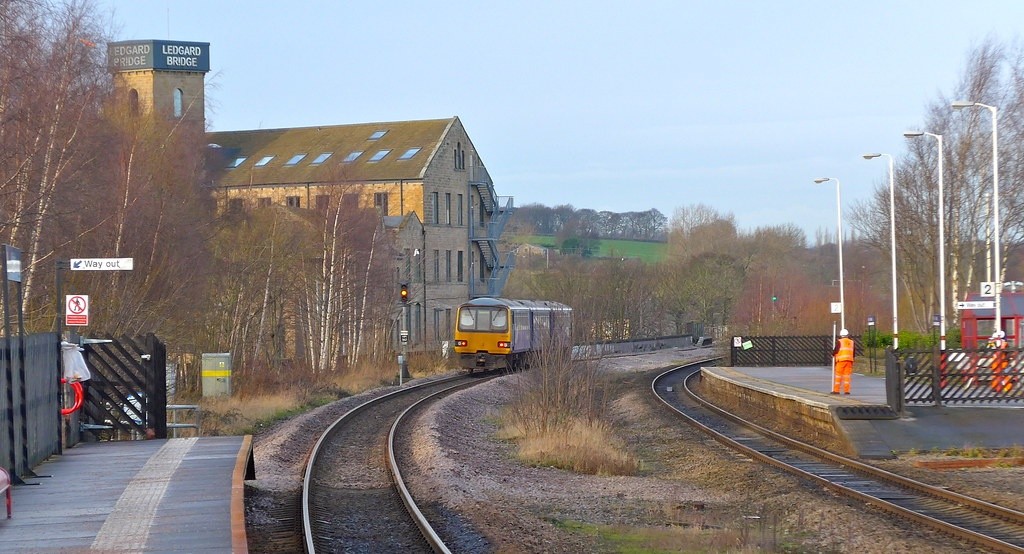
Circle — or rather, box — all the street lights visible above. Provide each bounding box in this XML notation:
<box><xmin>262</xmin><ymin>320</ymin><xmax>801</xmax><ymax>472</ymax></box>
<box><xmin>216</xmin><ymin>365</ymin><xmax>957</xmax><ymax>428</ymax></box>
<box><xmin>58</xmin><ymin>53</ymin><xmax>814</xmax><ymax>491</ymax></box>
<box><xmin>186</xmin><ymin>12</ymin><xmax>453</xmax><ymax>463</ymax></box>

<box><xmin>862</xmin><ymin>153</ymin><xmax>899</xmax><ymax>350</ymax></box>
<box><xmin>903</xmin><ymin>130</ymin><xmax>949</xmax><ymax>387</ymax></box>
<box><xmin>813</xmin><ymin>177</ymin><xmax>844</xmax><ymax>329</ymax></box>
<box><xmin>952</xmin><ymin>101</ymin><xmax>1004</xmax><ymax>394</ymax></box>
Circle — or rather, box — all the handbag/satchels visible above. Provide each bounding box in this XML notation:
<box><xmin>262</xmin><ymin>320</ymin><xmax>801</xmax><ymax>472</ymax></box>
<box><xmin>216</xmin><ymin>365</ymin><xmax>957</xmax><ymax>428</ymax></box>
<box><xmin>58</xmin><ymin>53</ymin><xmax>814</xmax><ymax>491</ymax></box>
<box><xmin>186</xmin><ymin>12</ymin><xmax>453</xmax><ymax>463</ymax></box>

<box><xmin>63</xmin><ymin>348</ymin><xmax>89</xmax><ymax>384</ymax></box>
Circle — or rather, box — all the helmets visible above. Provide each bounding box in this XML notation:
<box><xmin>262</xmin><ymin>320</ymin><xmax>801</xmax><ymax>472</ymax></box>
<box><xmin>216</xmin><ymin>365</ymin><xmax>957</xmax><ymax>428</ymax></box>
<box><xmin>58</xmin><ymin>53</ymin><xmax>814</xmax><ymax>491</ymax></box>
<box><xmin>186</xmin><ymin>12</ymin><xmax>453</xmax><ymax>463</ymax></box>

<box><xmin>999</xmin><ymin>331</ymin><xmax>1005</xmax><ymax>338</ymax></box>
<box><xmin>993</xmin><ymin>333</ymin><xmax>998</xmax><ymax>338</ymax></box>
<box><xmin>840</xmin><ymin>329</ymin><xmax>848</xmax><ymax>335</ymax></box>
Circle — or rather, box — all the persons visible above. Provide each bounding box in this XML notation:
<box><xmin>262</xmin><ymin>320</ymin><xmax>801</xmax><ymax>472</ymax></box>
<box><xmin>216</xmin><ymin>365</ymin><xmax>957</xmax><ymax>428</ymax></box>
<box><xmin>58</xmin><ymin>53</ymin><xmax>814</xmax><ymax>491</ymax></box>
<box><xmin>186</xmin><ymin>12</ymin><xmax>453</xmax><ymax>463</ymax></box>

<box><xmin>989</xmin><ymin>330</ymin><xmax>1024</xmax><ymax>398</ymax></box>
<box><xmin>830</xmin><ymin>329</ymin><xmax>855</xmax><ymax>396</ymax></box>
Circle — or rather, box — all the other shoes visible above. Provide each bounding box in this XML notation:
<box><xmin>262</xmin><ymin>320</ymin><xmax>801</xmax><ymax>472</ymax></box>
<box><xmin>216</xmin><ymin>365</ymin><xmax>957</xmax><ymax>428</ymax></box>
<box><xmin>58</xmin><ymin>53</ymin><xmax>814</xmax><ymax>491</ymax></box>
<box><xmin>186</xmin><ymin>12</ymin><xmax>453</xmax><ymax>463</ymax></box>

<box><xmin>831</xmin><ymin>392</ymin><xmax>839</xmax><ymax>394</ymax></box>
<box><xmin>845</xmin><ymin>392</ymin><xmax>850</xmax><ymax>394</ymax></box>
<box><xmin>992</xmin><ymin>389</ymin><xmax>995</xmax><ymax>392</ymax></box>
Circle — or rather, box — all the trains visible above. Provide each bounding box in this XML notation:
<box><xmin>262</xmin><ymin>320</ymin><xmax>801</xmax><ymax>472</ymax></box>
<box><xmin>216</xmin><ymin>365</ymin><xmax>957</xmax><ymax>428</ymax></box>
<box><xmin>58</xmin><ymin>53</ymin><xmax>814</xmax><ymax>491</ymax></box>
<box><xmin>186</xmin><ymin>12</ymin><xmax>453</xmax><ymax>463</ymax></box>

<box><xmin>451</xmin><ymin>295</ymin><xmax>575</xmax><ymax>375</ymax></box>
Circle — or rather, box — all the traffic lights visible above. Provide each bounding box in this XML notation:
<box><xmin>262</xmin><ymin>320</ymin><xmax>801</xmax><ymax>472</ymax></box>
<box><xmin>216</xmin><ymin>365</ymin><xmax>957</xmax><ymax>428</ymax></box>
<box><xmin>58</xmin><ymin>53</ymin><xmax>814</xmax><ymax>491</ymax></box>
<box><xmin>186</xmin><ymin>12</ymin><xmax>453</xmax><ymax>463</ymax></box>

<box><xmin>401</xmin><ymin>283</ymin><xmax>408</xmax><ymax>304</ymax></box>
<box><xmin>772</xmin><ymin>294</ymin><xmax>777</xmax><ymax>302</ymax></box>
